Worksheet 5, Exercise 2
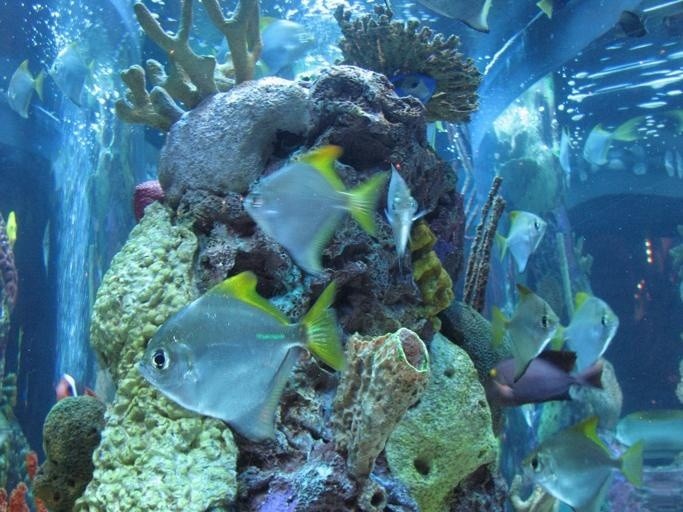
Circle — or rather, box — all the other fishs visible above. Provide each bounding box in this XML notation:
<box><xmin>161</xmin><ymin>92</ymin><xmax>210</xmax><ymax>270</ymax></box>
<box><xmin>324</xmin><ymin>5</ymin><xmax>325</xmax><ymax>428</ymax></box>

<box><xmin>522</xmin><ymin>409</ymin><xmax>646</xmax><ymax>512</ymax></box>
<box><xmin>5</xmin><ymin>210</ymin><xmax>17</xmax><ymax>249</ymax></box>
<box><xmin>216</xmin><ymin>17</ymin><xmax>316</xmax><ymax>76</ymax></box>
<box><xmin>383</xmin><ymin>163</ymin><xmax>431</xmax><ymax>259</ymax></box>
<box><xmin>583</xmin><ymin>116</ymin><xmax>645</xmax><ymax>166</ymax></box>
<box><xmin>485</xmin><ymin>283</ymin><xmax>619</xmax><ymax>407</ymax></box>
<box><xmin>559</xmin><ymin>125</ymin><xmax>574</xmax><ymax>174</ymax></box>
<box><xmin>243</xmin><ymin>145</ymin><xmax>390</xmax><ymax>280</ymax></box>
<box><xmin>495</xmin><ymin>210</ymin><xmax>548</xmax><ymax>273</ymax></box>
<box><xmin>7</xmin><ymin>60</ymin><xmax>44</xmax><ymax>119</ymax></box>
<box><xmin>47</xmin><ymin>42</ymin><xmax>96</xmax><ymax>107</ymax></box>
<box><xmin>134</xmin><ymin>271</ymin><xmax>349</xmax><ymax>443</ymax></box>
<box><xmin>415</xmin><ymin>0</ymin><xmax>494</xmax><ymax>34</ymax></box>
<box><xmin>54</xmin><ymin>373</ymin><xmax>97</xmax><ymax>403</ymax></box>
